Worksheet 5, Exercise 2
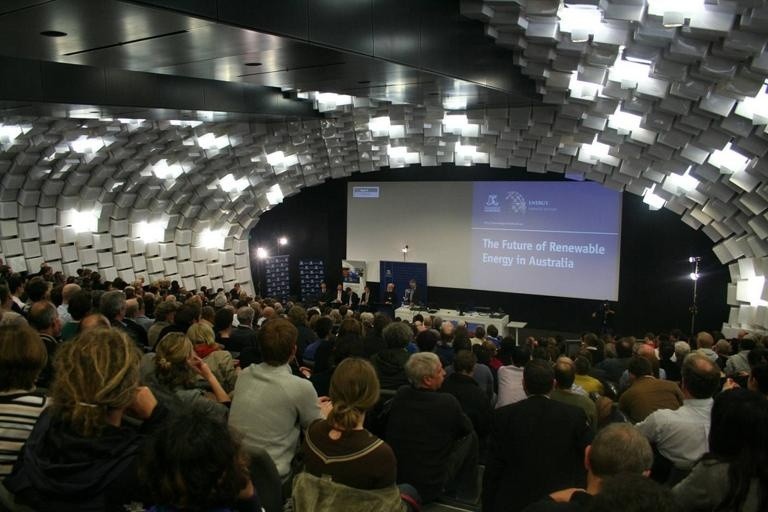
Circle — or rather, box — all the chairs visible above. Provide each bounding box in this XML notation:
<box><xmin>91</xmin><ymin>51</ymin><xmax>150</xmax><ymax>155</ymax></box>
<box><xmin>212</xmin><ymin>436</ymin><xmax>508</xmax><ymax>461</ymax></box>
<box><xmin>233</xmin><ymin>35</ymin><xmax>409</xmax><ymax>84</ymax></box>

<box><xmin>242</xmin><ymin>443</ymin><xmax>287</xmax><ymax>512</ymax></box>
<box><xmin>371</xmin><ymin>389</ymin><xmax>396</xmax><ymax>416</ymax></box>
<box><xmin>590</xmin><ymin>379</ymin><xmax>629</xmax><ymax>424</ymax></box>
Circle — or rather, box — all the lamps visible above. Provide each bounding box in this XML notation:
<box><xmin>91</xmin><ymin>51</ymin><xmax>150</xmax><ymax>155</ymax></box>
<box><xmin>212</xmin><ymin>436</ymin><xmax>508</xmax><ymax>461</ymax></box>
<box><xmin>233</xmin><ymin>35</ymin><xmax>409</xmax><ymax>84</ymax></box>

<box><xmin>402</xmin><ymin>245</ymin><xmax>408</xmax><ymax>253</ymax></box>
<box><xmin>689</xmin><ymin>256</ymin><xmax>701</xmax><ymax>333</ymax></box>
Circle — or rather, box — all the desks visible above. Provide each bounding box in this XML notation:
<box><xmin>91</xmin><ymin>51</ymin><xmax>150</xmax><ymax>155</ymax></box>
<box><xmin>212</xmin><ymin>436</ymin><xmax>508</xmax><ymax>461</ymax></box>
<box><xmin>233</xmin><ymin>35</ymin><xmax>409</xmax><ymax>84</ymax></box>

<box><xmin>394</xmin><ymin>305</ymin><xmax>509</xmax><ymax>339</ymax></box>
<box><xmin>506</xmin><ymin>321</ymin><xmax>527</xmax><ymax>346</ymax></box>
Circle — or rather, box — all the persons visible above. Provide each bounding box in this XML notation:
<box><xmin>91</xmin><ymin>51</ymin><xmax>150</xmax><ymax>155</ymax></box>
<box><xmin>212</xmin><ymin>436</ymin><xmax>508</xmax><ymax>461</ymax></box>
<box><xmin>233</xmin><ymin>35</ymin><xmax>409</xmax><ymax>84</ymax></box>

<box><xmin>489</xmin><ymin>303</ymin><xmax>768</xmax><ymax>478</ymax></box>
<box><xmin>1</xmin><ymin>313</ymin><xmax>54</xmax><ymax>482</ymax></box>
<box><xmin>126</xmin><ymin>390</ymin><xmax>264</xmax><ymax>512</ymax></box>
<box><xmin>671</xmin><ymin>387</ymin><xmax>767</xmax><ymax>512</ymax></box>
<box><xmin>4</xmin><ymin>327</ymin><xmax>166</xmax><ymax>510</ymax></box>
<box><xmin>304</xmin><ymin>356</ymin><xmax>397</xmax><ymax>490</ymax></box>
<box><xmin>590</xmin><ymin>472</ymin><xmax>683</xmax><ymax>511</ymax></box>
<box><xmin>381</xmin><ymin>352</ymin><xmax>478</xmax><ymax>502</ymax></box>
<box><xmin>226</xmin><ymin>317</ymin><xmax>332</xmax><ymax>502</ymax></box>
<box><xmin>1</xmin><ymin>263</ymin><xmax>315</xmax><ymax>405</ymax></box>
<box><xmin>480</xmin><ymin>357</ymin><xmax>592</xmax><ymax>510</ymax></box>
<box><xmin>526</xmin><ymin>420</ymin><xmax>654</xmax><ymax>511</ymax></box>
<box><xmin>308</xmin><ymin>278</ymin><xmax>516</xmax><ymax>396</ymax></box>
